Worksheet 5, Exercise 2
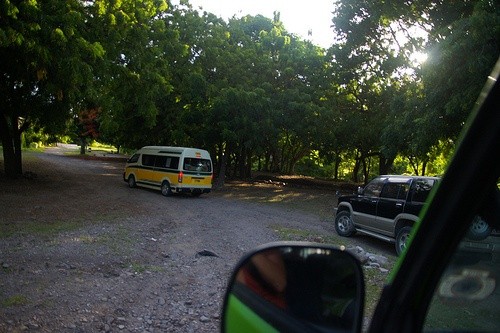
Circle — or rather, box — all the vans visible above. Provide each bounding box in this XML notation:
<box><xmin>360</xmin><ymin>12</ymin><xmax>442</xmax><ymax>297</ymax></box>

<box><xmin>123</xmin><ymin>145</ymin><xmax>213</xmax><ymax>196</ymax></box>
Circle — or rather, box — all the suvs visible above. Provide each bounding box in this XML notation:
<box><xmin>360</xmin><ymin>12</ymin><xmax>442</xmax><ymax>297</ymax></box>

<box><xmin>335</xmin><ymin>175</ymin><xmax>494</xmax><ymax>259</ymax></box>
